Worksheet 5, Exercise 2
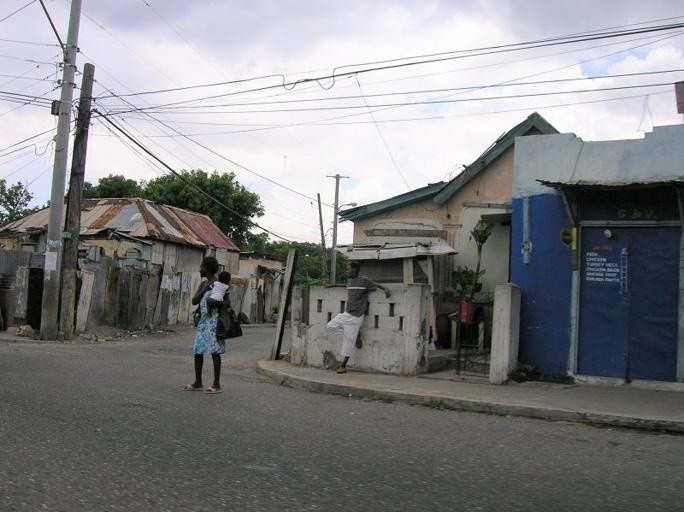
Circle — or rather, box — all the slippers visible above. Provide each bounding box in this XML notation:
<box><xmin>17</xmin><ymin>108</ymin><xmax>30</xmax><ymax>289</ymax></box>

<box><xmin>183</xmin><ymin>383</ymin><xmax>203</xmax><ymax>392</ymax></box>
<box><xmin>205</xmin><ymin>386</ymin><xmax>223</xmax><ymax>394</ymax></box>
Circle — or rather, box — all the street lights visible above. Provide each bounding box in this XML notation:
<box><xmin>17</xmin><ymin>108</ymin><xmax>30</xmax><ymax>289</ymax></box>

<box><xmin>329</xmin><ymin>201</ymin><xmax>358</xmax><ymax>282</ymax></box>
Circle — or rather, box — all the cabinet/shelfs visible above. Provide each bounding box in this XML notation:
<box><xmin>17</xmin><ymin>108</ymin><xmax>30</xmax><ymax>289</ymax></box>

<box><xmin>454</xmin><ymin>317</ymin><xmax>488</xmax><ymax>380</ymax></box>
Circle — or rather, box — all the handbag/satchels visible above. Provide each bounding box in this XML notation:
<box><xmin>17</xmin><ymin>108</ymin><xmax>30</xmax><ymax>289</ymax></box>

<box><xmin>193</xmin><ymin>309</ymin><xmax>201</xmax><ymax>326</ymax></box>
<box><xmin>216</xmin><ymin>308</ymin><xmax>244</xmax><ymax>340</ymax></box>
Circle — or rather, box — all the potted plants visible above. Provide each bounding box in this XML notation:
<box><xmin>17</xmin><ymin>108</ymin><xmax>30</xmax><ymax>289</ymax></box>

<box><xmin>451</xmin><ymin>262</ymin><xmax>487</xmax><ymax>326</ymax></box>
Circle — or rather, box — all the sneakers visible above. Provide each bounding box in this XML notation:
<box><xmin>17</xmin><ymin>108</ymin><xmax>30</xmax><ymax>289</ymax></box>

<box><xmin>337</xmin><ymin>366</ymin><xmax>347</xmax><ymax>373</ymax></box>
<box><xmin>355</xmin><ymin>331</ymin><xmax>362</xmax><ymax>348</ymax></box>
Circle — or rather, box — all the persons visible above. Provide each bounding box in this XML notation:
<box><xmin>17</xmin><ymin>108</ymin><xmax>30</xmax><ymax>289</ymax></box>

<box><xmin>324</xmin><ymin>263</ymin><xmax>391</xmax><ymax>374</ymax></box>
<box><xmin>206</xmin><ymin>272</ymin><xmax>231</xmax><ymax>317</ymax></box>
<box><xmin>182</xmin><ymin>257</ymin><xmax>230</xmax><ymax>394</ymax></box>
